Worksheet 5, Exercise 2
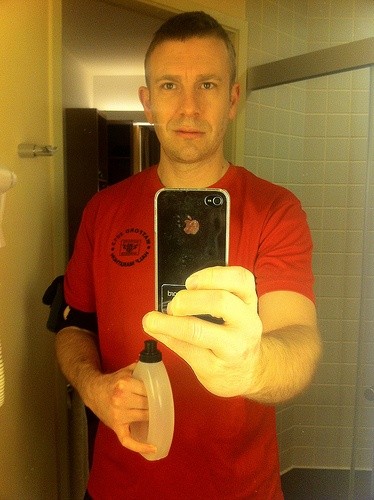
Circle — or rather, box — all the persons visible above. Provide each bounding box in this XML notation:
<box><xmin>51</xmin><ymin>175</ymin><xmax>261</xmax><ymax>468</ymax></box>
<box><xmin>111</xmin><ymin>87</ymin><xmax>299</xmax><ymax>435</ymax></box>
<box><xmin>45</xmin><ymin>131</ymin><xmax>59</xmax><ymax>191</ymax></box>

<box><xmin>42</xmin><ymin>10</ymin><xmax>321</xmax><ymax>500</ymax></box>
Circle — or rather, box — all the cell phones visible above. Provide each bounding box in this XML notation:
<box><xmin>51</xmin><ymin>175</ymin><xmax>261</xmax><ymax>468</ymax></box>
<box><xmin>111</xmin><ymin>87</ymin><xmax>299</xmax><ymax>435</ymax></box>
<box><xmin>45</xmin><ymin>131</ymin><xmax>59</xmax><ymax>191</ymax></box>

<box><xmin>154</xmin><ymin>187</ymin><xmax>230</xmax><ymax>326</ymax></box>
